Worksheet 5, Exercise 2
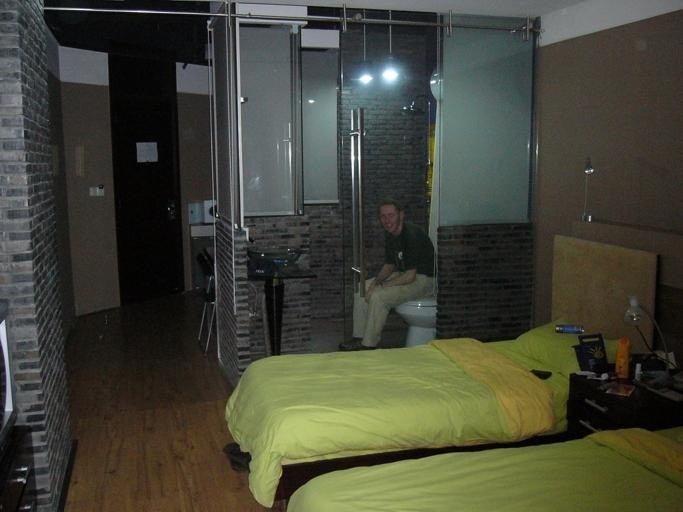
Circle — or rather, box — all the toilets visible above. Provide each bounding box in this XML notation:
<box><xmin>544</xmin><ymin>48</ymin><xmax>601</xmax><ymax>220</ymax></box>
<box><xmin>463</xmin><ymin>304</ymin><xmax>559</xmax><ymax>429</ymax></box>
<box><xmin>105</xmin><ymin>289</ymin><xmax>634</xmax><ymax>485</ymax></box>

<box><xmin>395</xmin><ymin>296</ymin><xmax>437</xmax><ymax>347</ymax></box>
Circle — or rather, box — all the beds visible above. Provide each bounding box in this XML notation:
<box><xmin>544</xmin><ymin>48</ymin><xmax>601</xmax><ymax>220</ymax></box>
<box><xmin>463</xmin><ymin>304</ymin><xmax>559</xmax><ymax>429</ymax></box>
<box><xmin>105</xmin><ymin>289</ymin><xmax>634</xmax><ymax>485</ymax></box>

<box><xmin>224</xmin><ymin>232</ymin><xmax>658</xmax><ymax>507</ymax></box>
<box><xmin>286</xmin><ymin>426</ymin><xmax>682</xmax><ymax>512</ymax></box>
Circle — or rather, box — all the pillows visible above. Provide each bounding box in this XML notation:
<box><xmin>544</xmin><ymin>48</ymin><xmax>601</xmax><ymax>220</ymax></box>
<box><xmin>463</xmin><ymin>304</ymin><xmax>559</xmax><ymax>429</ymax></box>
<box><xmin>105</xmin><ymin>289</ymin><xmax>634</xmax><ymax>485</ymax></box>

<box><xmin>510</xmin><ymin>313</ymin><xmax>618</xmax><ymax>381</ymax></box>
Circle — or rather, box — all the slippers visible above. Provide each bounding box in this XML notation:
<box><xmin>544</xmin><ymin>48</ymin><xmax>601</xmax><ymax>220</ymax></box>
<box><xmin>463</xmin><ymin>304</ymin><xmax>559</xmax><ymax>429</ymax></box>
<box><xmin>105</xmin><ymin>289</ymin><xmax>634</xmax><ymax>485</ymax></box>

<box><xmin>224</xmin><ymin>441</ymin><xmax>251</xmax><ymax>472</ymax></box>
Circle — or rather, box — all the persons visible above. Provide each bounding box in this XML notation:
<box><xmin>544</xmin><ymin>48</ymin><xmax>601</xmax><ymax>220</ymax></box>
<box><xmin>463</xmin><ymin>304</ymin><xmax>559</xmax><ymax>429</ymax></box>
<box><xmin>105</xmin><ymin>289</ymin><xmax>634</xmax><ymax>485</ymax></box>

<box><xmin>338</xmin><ymin>198</ymin><xmax>437</xmax><ymax>351</ymax></box>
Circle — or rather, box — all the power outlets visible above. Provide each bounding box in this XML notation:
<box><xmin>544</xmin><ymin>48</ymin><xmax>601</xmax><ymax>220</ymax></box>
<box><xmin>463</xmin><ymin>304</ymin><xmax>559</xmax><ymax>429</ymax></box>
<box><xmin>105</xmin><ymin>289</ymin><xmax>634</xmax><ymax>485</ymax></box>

<box><xmin>203</xmin><ymin>200</ymin><xmax>220</xmax><ymax>224</ymax></box>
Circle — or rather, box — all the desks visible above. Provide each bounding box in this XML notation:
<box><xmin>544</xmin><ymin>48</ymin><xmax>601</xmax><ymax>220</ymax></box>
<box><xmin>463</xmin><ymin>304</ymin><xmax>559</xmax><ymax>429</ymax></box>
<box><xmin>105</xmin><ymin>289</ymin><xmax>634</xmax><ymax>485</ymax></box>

<box><xmin>245</xmin><ymin>261</ymin><xmax>316</xmax><ymax>356</ymax></box>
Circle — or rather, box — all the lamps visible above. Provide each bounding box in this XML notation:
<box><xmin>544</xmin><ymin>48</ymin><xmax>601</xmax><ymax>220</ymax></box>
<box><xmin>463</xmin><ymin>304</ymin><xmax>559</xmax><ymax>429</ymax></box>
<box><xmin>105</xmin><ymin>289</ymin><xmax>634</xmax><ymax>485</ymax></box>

<box><xmin>621</xmin><ymin>295</ymin><xmax>669</xmax><ymax>374</ymax></box>
<box><xmin>579</xmin><ymin>157</ymin><xmax>594</xmax><ymax>224</ymax></box>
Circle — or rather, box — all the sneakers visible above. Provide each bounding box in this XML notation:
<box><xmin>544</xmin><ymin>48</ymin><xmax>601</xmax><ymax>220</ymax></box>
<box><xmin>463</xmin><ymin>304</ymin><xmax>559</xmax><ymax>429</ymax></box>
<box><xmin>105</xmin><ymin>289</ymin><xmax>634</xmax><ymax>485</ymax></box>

<box><xmin>338</xmin><ymin>337</ymin><xmax>362</xmax><ymax>350</ymax></box>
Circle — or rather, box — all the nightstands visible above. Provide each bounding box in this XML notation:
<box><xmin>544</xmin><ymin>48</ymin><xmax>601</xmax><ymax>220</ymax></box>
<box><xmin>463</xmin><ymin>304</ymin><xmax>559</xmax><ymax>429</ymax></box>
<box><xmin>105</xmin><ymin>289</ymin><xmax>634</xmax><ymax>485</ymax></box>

<box><xmin>565</xmin><ymin>369</ymin><xmax>681</xmax><ymax>439</ymax></box>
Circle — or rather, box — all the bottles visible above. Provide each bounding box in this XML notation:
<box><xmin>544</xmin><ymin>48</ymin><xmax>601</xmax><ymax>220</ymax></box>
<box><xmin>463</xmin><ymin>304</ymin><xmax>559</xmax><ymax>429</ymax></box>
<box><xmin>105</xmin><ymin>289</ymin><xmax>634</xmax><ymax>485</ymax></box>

<box><xmin>634</xmin><ymin>363</ymin><xmax>641</xmax><ymax>381</ymax></box>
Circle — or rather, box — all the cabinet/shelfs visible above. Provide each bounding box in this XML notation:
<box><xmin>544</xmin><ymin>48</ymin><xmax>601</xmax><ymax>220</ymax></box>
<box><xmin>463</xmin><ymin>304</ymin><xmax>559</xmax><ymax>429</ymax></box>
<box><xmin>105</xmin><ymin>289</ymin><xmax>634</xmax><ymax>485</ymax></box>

<box><xmin>0</xmin><ymin>425</ymin><xmax>38</xmax><ymax>512</ymax></box>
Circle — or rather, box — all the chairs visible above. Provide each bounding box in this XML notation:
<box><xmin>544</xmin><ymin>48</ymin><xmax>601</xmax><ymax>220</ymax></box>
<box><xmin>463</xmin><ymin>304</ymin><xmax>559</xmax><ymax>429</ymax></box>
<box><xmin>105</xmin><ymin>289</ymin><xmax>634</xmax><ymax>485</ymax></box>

<box><xmin>196</xmin><ymin>253</ymin><xmax>215</xmax><ymax>357</ymax></box>
